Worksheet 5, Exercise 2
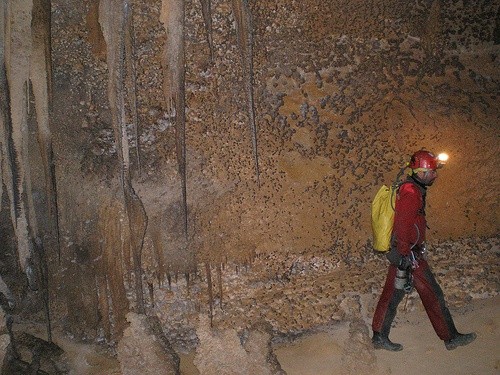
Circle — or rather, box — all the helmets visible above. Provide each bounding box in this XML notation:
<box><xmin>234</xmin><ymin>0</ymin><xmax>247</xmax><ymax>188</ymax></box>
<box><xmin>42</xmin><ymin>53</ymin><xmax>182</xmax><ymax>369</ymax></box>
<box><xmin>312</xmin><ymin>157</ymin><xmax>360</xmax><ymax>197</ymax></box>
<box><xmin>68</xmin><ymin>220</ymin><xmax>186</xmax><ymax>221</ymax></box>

<box><xmin>409</xmin><ymin>150</ymin><xmax>439</xmax><ymax>173</ymax></box>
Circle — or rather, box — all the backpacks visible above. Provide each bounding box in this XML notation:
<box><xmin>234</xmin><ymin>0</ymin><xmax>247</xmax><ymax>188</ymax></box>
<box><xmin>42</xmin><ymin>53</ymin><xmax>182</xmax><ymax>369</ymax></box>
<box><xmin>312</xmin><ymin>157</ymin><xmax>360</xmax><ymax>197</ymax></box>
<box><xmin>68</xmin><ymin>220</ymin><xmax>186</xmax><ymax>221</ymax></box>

<box><xmin>371</xmin><ymin>180</ymin><xmax>427</xmax><ymax>254</ymax></box>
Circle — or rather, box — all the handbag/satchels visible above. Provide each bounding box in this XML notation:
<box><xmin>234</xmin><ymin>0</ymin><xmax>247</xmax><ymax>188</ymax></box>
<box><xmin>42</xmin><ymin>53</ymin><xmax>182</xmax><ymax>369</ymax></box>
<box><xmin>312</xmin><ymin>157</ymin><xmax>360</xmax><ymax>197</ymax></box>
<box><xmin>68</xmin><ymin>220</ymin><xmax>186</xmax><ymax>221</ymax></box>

<box><xmin>385</xmin><ymin>247</ymin><xmax>406</xmax><ymax>267</ymax></box>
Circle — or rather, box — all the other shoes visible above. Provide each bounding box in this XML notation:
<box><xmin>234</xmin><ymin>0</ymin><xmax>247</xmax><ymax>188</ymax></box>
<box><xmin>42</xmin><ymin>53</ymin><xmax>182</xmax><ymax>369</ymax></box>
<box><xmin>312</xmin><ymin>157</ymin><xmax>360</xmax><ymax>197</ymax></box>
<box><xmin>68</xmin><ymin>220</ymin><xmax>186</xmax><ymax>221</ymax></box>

<box><xmin>444</xmin><ymin>332</ymin><xmax>478</xmax><ymax>349</ymax></box>
<box><xmin>371</xmin><ymin>332</ymin><xmax>404</xmax><ymax>351</ymax></box>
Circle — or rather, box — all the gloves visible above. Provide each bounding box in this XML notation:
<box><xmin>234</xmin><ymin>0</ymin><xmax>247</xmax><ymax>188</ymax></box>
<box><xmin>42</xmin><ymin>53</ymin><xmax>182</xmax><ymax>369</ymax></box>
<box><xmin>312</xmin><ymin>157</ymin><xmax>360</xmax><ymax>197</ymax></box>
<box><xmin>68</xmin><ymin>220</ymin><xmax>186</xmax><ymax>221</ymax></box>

<box><xmin>396</xmin><ymin>256</ymin><xmax>413</xmax><ymax>271</ymax></box>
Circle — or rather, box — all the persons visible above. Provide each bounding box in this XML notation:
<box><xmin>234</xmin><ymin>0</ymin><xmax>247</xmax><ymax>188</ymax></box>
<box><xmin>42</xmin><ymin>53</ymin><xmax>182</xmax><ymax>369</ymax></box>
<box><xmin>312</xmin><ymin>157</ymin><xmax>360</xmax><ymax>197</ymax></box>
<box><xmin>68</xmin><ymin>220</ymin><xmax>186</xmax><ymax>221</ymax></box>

<box><xmin>371</xmin><ymin>147</ymin><xmax>477</xmax><ymax>352</ymax></box>
